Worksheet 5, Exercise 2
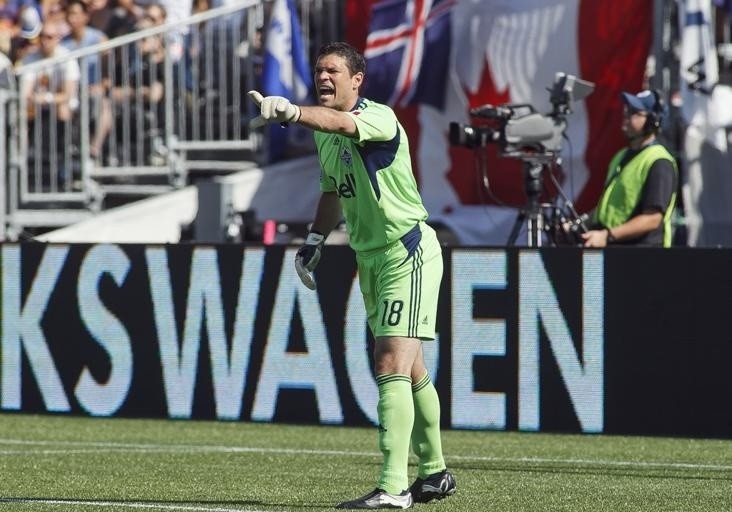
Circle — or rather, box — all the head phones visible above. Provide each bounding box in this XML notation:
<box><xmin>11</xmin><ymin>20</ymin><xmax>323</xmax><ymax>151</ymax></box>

<box><xmin>646</xmin><ymin>88</ymin><xmax>664</xmax><ymax>131</ymax></box>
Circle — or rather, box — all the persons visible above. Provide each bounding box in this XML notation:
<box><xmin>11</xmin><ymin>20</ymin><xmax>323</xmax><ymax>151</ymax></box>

<box><xmin>247</xmin><ymin>41</ymin><xmax>455</xmax><ymax>509</ymax></box>
<box><xmin>0</xmin><ymin>1</ymin><xmax>268</xmax><ymax>210</ymax></box>
<box><xmin>564</xmin><ymin>86</ymin><xmax>680</xmax><ymax>249</ymax></box>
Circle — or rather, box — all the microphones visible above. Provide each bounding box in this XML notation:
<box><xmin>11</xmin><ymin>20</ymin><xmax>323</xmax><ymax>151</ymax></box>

<box><xmin>626</xmin><ymin>130</ymin><xmax>652</xmax><ymax>141</ymax></box>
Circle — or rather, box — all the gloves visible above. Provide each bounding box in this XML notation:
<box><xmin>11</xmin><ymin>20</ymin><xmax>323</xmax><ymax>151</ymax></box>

<box><xmin>247</xmin><ymin>90</ymin><xmax>301</xmax><ymax>130</ymax></box>
<box><xmin>295</xmin><ymin>230</ymin><xmax>327</xmax><ymax>290</ymax></box>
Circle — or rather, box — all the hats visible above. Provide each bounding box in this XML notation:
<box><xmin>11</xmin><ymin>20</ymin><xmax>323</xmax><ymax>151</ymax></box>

<box><xmin>19</xmin><ymin>6</ymin><xmax>42</xmax><ymax>40</ymax></box>
<box><xmin>623</xmin><ymin>90</ymin><xmax>669</xmax><ymax>126</ymax></box>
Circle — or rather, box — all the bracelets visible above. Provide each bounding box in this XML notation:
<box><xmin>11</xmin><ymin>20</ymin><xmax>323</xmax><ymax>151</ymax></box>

<box><xmin>602</xmin><ymin>224</ymin><xmax>618</xmax><ymax>246</ymax></box>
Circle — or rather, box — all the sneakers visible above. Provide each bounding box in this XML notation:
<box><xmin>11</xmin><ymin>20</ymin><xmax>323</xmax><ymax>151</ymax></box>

<box><xmin>337</xmin><ymin>488</ymin><xmax>415</xmax><ymax>509</ymax></box>
<box><xmin>409</xmin><ymin>470</ymin><xmax>458</xmax><ymax>503</ymax></box>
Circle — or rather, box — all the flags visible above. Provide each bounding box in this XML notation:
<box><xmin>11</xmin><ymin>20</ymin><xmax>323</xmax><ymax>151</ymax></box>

<box><xmin>676</xmin><ymin>0</ymin><xmax>729</xmax><ymax>157</ymax></box>
<box><xmin>354</xmin><ymin>1</ymin><xmax>456</xmax><ymax>113</ymax></box>
<box><xmin>260</xmin><ymin>2</ymin><xmax>317</xmax><ymax>161</ymax></box>
